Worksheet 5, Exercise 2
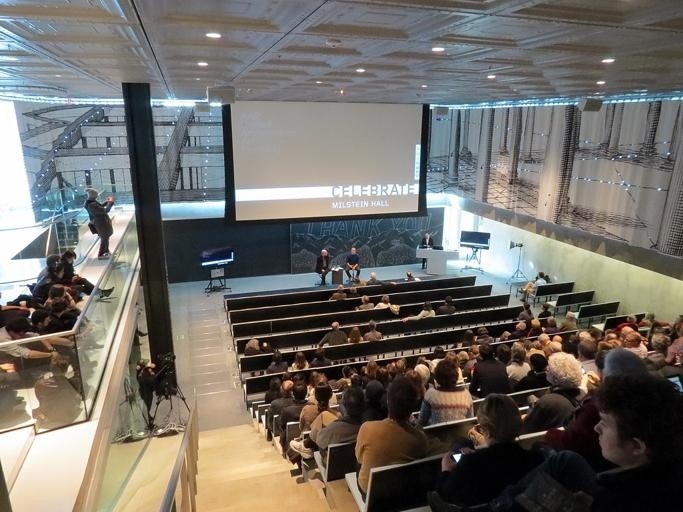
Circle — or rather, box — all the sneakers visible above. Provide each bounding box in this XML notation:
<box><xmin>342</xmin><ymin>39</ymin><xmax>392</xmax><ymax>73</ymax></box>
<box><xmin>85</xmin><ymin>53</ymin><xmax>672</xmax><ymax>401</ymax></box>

<box><xmin>99</xmin><ymin>286</ymin><xmax>115</xmax><ymax>298</ymax></box>
<box><xmin>289</xmin><ymin>440</ymin><xmax>314</xmax><ymax>459</ymax></box>
<box><xmin>98</xmin><ymin>252</ymin><xmax>112</xmax><ymax>259</ymax></box>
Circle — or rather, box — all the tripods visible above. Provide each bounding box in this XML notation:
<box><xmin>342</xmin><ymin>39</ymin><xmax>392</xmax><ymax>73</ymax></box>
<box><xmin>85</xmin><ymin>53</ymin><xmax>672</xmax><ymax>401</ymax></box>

<box><xmin>151</xmin><ymin>384</ymin><xmax>190</xmax><ymax>425</ymax></box>
<box><xmin>506</xmin><ymin>247</ymin><xmax>529</xmax><ymax>284</ymax></box>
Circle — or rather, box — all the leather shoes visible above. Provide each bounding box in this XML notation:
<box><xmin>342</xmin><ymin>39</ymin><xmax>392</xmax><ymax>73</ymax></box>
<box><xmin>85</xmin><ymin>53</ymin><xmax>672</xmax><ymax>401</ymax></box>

<box><xmin>427</xmin><ymin>491</ymin><xmax>461</xmax><ymax>511</ymax></box>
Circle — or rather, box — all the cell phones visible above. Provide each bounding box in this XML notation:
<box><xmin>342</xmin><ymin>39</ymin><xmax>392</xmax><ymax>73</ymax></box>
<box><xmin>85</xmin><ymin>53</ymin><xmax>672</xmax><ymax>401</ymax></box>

<box><xmin>452</xmin><ymin>453</ymin><xmax>461</xmax><ymax>463</ymax></box>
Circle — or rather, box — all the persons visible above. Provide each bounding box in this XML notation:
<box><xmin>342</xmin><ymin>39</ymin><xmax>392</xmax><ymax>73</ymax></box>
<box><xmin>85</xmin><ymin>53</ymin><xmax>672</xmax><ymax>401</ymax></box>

<box><xmin>0</xmin><ymin>248</ymin><xmax>115</xmax><ymax>428</ymax></box>
<box><xmin>344</xmin><ymin>247</ymin><xmax>360</xmax><ymax>281</ymax></box>
<box><xmin>243</xmin><ymin>271</ymin><xmax>683</xmax><ymax>511</ymax></box>
<box><xmin>135</xmin><ymin>357</ymin><xmax>156</xmax><ymax>419</ymax></box>
<box><xmin>83</xmin><ymin>187</ymin><xmax>115</xmax><ymax>260</ymax></box>
<box><xmin>421</xmin><ymin>232</ymin><xmax>433</xmax><ymax>269</ymax></box>
<box><xmin>314</xmin><ymin>249</ymin><xmax>332</xmax><ymax>287</ymax></box>
<box><xmin>133</xmin><ymin>329</ymin><xmax>148</xmax><ymax>346</ymax></box>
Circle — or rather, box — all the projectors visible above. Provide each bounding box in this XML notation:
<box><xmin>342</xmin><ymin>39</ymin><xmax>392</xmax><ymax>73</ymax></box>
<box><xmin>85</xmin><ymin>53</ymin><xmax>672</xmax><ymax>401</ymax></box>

<box><xmin>434</xmin><ymin>107</ymin><xmax>449</xmax><ymax>115</ymax></box>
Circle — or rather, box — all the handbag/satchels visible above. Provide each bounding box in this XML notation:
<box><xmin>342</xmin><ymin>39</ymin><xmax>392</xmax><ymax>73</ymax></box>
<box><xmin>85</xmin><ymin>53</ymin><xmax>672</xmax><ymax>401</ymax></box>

<box><xmin>88</xmin><ymin>222</ymin><xmax>98</xmax><ymax>234</ymax></box>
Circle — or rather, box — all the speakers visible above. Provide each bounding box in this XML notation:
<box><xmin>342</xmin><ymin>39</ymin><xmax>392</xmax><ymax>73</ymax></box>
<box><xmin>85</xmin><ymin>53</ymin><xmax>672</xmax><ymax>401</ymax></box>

<box><xmin>207</xmin><ymin>85</ymin><xmax>235</xmax><ymax>104</ymax></box>
<box><xmin>578</xmin><ymin>98</ymin><xmax>602</xmax><ymax>112</ymax></box>
<box><xmin>195</xmin><ymin>102</ymin><xmax>210</xmax><ymax>112</ymax></box>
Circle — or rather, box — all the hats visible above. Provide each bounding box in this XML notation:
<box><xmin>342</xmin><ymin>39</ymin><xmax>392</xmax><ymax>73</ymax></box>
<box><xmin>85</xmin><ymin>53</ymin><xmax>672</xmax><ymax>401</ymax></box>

<box><xmin>84</xmin><ymin>188</ymin><xmax>99</xmax><ymax>199</ymax></box>
